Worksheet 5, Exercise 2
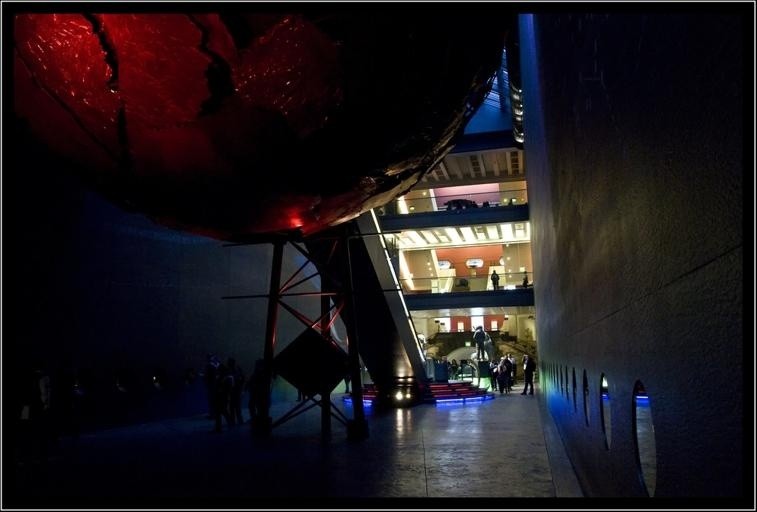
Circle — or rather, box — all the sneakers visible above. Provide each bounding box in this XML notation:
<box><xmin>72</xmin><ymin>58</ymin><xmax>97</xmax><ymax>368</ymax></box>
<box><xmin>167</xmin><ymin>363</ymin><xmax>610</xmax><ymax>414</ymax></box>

<box><xmin>521</xmin><ymin>392</ymin><xmax>535</xmax><ymax>396</ymax></box>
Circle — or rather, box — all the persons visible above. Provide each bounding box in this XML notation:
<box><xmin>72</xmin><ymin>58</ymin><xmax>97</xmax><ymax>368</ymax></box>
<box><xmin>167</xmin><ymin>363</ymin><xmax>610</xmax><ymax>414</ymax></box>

<box><xmin>523</xmin><ymin>271</ymin><xmax>528</xmax><ymax>288</ymax></box>
<box><xmin>296</xmin><ymin>389</ymin><xmax>306</xmax><ymax>401</ymax></box>
<box><xmin>202</xmin><ymin>355</ymin><xmax>272</xmax><ymax>434</ymax></box>
<box><xmin>491</xmin><ymin>270</ymin><xmax>500</xmax><ymax>290</ymax></box>
<box><xmin>441</xmin><ymin>356</ymin><xmax>474</xmax><ymax>381</ymax></box>
<box><xmin>473</xmin><ymin>326</ymin><xmax>489</xmax><ymax>359</ymax></box>
<box><xmin>521</xmin><ymin>352</ymin><xmax>536</xmax><ymax>395</ymax></box>
<box><xmin>489</xmin><ymin>353</ymin><xmax>517</xmax><ymax>394</ymax></box>
